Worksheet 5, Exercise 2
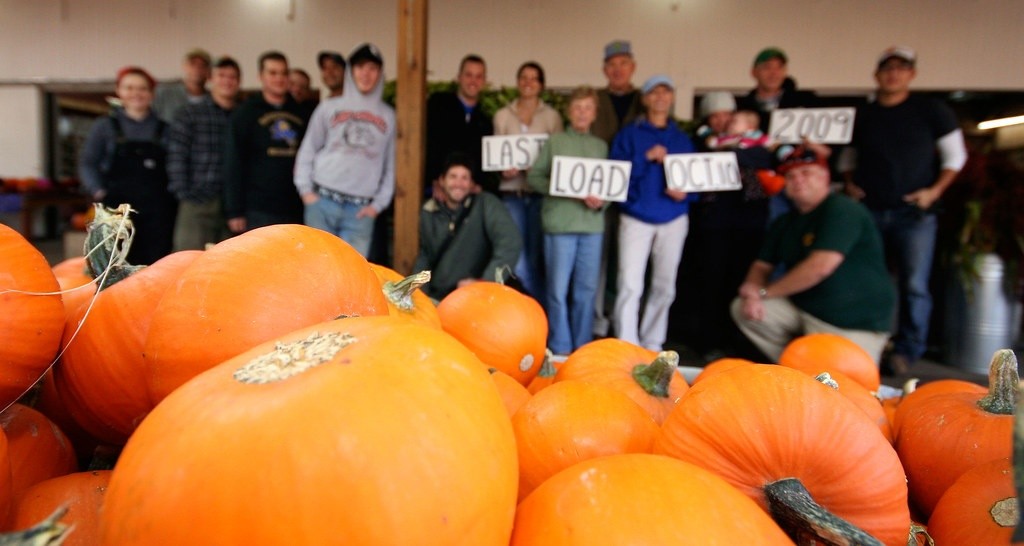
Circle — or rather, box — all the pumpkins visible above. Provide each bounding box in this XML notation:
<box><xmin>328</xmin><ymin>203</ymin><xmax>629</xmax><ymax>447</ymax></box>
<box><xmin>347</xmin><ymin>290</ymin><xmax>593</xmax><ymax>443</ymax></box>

<box><xmin>0</xmin><ymin>201</ymin><xmax>1024</xmax><ymax>546</ymax></box>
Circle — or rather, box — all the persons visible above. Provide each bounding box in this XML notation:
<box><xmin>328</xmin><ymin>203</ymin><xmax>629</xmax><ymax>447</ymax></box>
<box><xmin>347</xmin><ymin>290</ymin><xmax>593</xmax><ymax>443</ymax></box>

<box><xmin>836</xmin><ymin>40</ymin><xmax>969</xmax><ymax>372</ymax></box>
<box><xmin>612</xmin><ymin>73</ymin><xmax>702</xmax><ymax>349</ymax></box>
<box><xmin>729</xmin><ymin>147</ymin><xmax>905</xmax><ymax>400</ymax></box>
<box><xmin>687</xmin><ymin>88</ymin><xmax>776</xmax><ymax>360</ymax></box>
<box><xmin>74</xmin><ymin>42</ymin><xmax>399</xmax><ymax>266</ymax></box>
<box><xmin>739</xmin><ymin>47</ymin><xmax>825</xmax><ymax>133</ymax></box>
<box><xmin>426</xmin><ymin>53</ymin><xmax>499</xmax><ymax>202</ymax></box>
<box><xmin>589</xmin><ymin>38</ymin><xmax>648</xmax><ymax>337</ymax></box>
<box><xmin>525</xmin><ymin>85</ymin><xmax>608</xmax><ymax>355</ymax></box>
<box><xmin>409</xmin><ymin>165</ymin><xmax>525</xmax><ymax>300</ymax></box>
<box><xmin>494</xmin><ymin>61</ymin><xmax>563</xmax><ymax>298</ymax></box>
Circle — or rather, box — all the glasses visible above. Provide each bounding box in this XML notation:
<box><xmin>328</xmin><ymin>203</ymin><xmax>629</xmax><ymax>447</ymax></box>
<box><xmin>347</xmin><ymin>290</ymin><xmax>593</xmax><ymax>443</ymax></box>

<box><xmin>784</xmin><ymin>150</ymin><xmax>819</xmax><ymax>161</ymax></box>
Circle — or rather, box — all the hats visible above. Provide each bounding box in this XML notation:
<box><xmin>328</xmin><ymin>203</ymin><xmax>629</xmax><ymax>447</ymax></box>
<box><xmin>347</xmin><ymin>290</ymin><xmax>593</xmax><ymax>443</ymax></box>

<box><xmin>643</xmin><ymin>74</ymin><xmax>675</xmax><ymax>96</ymax></box>
<box><xmin>351</xmin><ymin>43</ymin><xmax>382</xmax><ymax>65</ymax></box>
<box><xmin>184</xmin><ymin>47</ymin><xmax>211</xmax><ymax>65</ymax></box>
<box><xmin>753</xmin><ymin>47</ymin><xmax>787</xmax><ymax>68</ymax></box>
<box><xmin>604</xmin><ymin>39</ymin><xmax>632</xmax><ymax>60</ymax></box>
<box><xmin>779</xmin><ymin>142</ymin><xmax>831</xmax><ymax>174</ymax></box>
<box><xmin>701</xmin><ymin>90</ymin><xmax>735</xmax><ymax>119</ymax></box>
<box><xmin>877</xmin><ymin>49</ymin><xmax>916</xmax><ymax>70</ymax></box>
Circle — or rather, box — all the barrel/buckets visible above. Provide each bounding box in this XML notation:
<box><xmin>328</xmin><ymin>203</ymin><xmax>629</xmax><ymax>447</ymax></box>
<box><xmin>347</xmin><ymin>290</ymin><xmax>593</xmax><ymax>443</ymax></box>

<box><xmin>961</xmin><ymin>257</ymin><xmax>1022</xmax><ymax>374</ymax></box>
<box><xmin>0</xmin><ymin>195</ymin><xmax>21</xmax><ymax>231</ymax></box>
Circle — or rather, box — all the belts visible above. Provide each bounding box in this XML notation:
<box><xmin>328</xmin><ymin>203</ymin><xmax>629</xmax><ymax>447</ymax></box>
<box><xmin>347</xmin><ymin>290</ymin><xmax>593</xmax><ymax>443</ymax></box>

<box><xmin>316</xmin><ymin>186</ymin><xmax>367</xmax><ymax>205</ymax></box>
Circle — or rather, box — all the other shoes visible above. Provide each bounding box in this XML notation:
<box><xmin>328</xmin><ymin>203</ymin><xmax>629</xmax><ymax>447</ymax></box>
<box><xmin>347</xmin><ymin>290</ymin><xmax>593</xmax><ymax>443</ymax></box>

<box><xmin>883</xmin><ymin>353</ymin><xmax>907</xmax><ymax>375</ymax></box>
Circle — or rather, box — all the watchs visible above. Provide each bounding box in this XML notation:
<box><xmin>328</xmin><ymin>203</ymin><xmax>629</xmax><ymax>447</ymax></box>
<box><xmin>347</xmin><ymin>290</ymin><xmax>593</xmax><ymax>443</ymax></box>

<box><xmin>760</xmin><ymin>289</ymin><xmax>766</xmax><ymax>296</ymax></box>
